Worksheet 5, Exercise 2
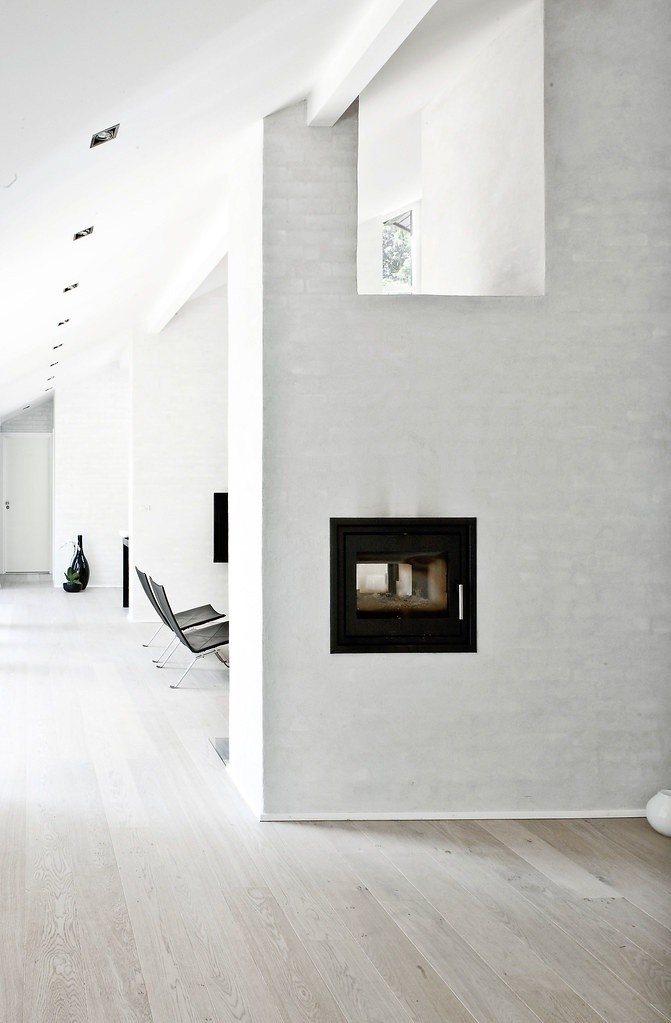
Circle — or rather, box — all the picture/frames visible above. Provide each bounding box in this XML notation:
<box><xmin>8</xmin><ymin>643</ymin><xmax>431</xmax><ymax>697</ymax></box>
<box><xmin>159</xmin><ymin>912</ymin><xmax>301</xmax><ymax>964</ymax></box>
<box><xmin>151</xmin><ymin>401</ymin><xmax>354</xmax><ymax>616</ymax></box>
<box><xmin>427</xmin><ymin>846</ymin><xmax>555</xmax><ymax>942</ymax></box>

<box><xmin>375</xmin><ymin>198</ymin><xmax>422</xmax><ymax>292</ymax></box>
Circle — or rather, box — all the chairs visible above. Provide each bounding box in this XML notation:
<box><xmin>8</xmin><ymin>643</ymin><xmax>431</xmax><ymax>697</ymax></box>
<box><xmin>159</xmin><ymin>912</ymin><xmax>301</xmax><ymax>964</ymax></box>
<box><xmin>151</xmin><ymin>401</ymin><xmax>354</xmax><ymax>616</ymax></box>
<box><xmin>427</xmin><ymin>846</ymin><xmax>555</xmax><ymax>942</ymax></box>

<box><xmin>136</xmin><ymin>566</ymin><xmax>229</xmax><ymax>689</ymax></box>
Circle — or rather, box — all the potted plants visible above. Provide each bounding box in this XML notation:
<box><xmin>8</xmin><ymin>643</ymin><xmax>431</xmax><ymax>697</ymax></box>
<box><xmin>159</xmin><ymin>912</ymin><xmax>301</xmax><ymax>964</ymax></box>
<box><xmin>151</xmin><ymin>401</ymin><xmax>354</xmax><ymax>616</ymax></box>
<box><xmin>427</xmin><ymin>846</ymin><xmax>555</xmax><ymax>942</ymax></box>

<box><xmin>63</xmin><ymin>567</ymin><xmax>82</xmax><ymax>592</ymax></box>
